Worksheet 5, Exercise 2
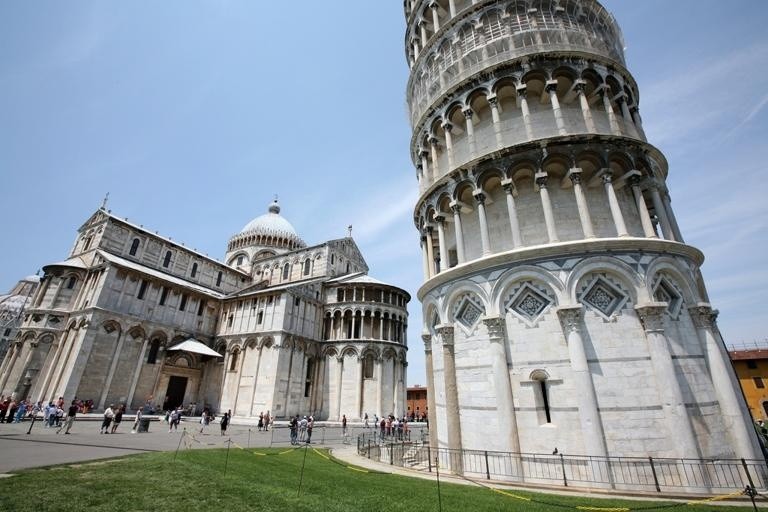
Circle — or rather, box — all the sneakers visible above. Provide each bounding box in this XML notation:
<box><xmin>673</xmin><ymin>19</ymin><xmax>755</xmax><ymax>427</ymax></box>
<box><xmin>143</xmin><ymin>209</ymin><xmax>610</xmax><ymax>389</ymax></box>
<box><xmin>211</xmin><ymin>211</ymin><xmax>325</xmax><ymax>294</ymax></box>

<box><xmin>0</xmin><ymin>413</ymin><xmax>138</xmax><ymax>435</ymax></box>
<box><xmin>259</xmin><ymin>425</ymin><xmax>378</xmax><ymax>446</ymax></box>
<box><xmin>161</xmin><ymin>420</ymin><xmax>227</xmax><ymax>438</ymax></box>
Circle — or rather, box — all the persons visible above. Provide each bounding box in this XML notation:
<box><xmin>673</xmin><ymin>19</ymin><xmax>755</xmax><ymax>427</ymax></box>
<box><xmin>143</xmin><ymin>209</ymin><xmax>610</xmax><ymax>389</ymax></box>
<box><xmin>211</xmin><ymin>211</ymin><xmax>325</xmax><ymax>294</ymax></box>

<box><xmin>255</xmin><ymin>409</ymin><xmax>430</xmax><ymax>446</ymax></box>
<box><xmin>755</xmin><ymin>418</ymin><xmax>764</xmax><ymax>430</ymax></box>
<box><xmin>0</xmin><ymin>392</ymin><xmax>233</xmax><ymax>437</ymax></box>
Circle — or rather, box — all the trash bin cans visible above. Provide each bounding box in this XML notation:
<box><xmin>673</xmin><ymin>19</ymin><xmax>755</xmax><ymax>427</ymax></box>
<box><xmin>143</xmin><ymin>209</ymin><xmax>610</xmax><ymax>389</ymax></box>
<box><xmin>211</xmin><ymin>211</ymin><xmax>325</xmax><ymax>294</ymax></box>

<box><xmin>137</xmin><ymin>417</ymin><xmax>150</xmax><ymax>432</ymax></box>
